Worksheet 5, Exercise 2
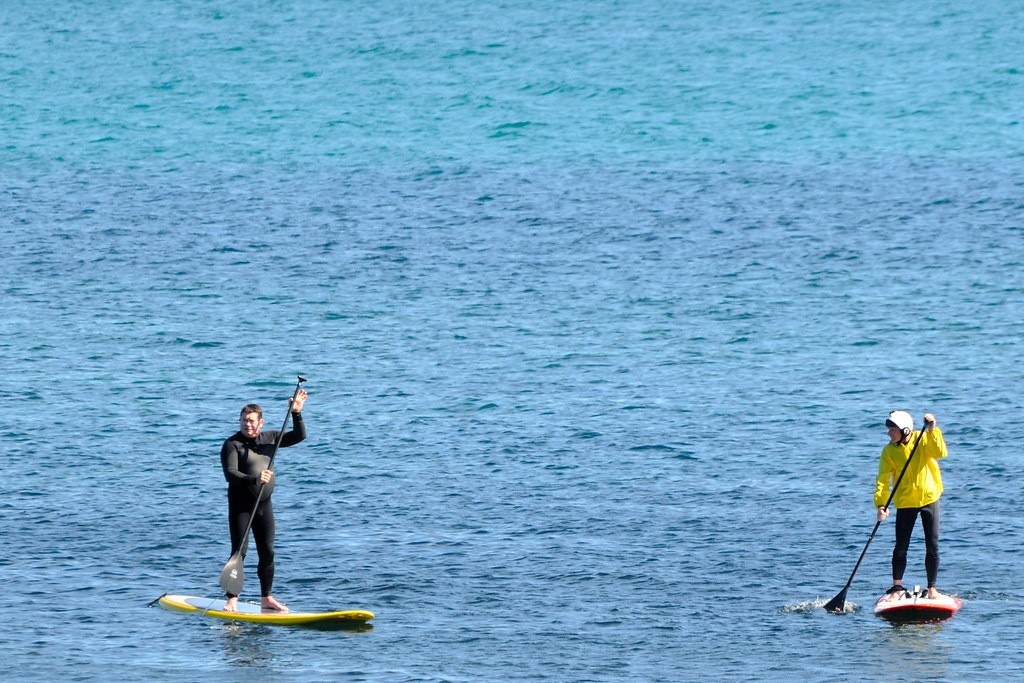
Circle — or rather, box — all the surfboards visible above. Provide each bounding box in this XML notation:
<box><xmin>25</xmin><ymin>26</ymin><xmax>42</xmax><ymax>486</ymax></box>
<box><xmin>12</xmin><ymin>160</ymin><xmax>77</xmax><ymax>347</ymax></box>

<box><xmin>873</xmin><ymin>587</ymin><xmax>956</xmax><ymax>613</ymax></box>
<box><xmin>159</xmin><ymin>594</ymin><xmax>375</xmax><ymax>625</ymax></box>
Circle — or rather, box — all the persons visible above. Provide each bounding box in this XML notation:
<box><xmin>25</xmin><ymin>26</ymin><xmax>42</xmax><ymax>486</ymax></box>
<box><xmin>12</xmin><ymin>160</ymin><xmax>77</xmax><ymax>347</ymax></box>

<box><xmin>873</xmin><ymin>409</ymin><xmax>947</xmax><ymax>604</ymax></box>
<box><xmin>220</xmin><ymin>387</ymin><xmax>309</xmax><ymax>613</ymax></box>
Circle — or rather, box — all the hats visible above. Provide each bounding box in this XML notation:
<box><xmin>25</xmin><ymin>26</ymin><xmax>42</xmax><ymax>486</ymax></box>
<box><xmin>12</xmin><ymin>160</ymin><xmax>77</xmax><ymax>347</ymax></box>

<box><xmin>887</xmin><ymin>410</ymin><xmax>913</xmax><ymax>436</ymax></box>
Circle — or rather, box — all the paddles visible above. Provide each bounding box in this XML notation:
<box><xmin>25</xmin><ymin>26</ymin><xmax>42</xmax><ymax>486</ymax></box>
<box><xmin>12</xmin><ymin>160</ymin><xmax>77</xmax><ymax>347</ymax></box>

<box><xmin>823</xmin><ymin>418</ymin><xmax>929</xmax><ymax>614</ymax></box>
<box><xmin>220</xmin><ymin>373</ymin><xmax>308</xmax><ymax>596</ymax></box>
